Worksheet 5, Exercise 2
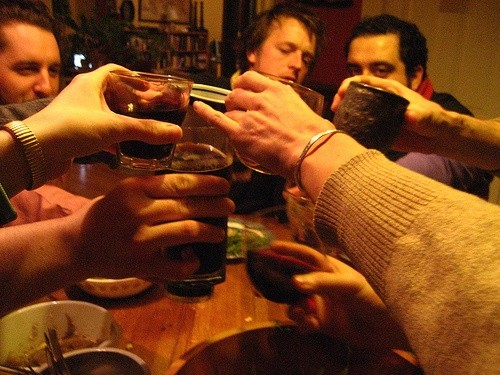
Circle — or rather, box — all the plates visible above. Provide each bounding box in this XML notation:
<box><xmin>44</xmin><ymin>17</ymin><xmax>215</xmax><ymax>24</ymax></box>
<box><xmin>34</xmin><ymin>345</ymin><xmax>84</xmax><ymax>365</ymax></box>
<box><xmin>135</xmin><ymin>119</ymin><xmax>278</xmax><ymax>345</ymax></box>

<box><xmin>226</xmin><ymin>217</ymin><xmax>272</xmax><ymax>259</ymax></box>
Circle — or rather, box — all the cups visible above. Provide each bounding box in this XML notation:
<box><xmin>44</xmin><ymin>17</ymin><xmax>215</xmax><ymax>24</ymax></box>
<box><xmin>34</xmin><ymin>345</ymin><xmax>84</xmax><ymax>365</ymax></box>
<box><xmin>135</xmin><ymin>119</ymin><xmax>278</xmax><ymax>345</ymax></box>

<box><xmin>235</xmin><ymin>71</ymin><xmax>324</xmax><ymax>175</ymax></box>
<box><xmin>242</xmin><ymin>206</ymin><xmax>327</xmax><ymax>304</ymax></box>
<box><xmin>334</xmin><ymin>81</ymin><xmax>409</xmax><ymax>156</ymax></box>
<box><xmin>180</xmin><ymin>83</ymin><xmax>232</xmax><ymax>150</ymax></box>
<box><xmin>160</xmin><ymin>141</ymin><xmax>234</xmax><ymax>285</ymax></box>
<box><xmin>107</xmin><ymin>69</ymin><xmax>193</xmax><ymax>171</ymax></box>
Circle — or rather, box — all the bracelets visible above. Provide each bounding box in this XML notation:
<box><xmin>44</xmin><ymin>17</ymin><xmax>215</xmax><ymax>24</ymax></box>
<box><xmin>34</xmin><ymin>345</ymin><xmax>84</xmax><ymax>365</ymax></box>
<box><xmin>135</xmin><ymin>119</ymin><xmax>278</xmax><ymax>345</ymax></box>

<box><xmin>294</xmin><ymin>129</ymin><xmax>347</xmax><ymax>194</ymax></box>
<box><xmin>0</xmin><ymin>120</ymin><xmax>46</xmax><ymax>190</ymax></box>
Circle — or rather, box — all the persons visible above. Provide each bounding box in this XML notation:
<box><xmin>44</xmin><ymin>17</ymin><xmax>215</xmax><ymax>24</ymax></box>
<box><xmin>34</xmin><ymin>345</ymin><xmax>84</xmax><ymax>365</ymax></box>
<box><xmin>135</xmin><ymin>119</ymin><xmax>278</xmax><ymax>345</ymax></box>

<box><xmin>0</xmin><ymin>0</ymin><xmax>500</xmax><ymax>375</ymax></box>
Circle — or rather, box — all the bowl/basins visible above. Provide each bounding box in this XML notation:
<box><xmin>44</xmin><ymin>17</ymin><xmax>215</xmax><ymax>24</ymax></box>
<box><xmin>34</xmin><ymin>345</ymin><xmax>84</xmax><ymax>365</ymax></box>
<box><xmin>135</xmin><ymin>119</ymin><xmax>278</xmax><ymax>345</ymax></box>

<box><xmin>165</xmin><ymin>319</ymin><xmax>423</xmax><ymax>375</ymax></box>
<box><xmin>0</xmin><ymin>300</ymin><xmax>116</xmax><ymax>371</ymax></box>
<box><xmin>34</xmin><ymin>347</ymin><xmax>150</xmax><ymax>375</ymax></box>
<box><xmin>75</xmin><ymin>277</ymin><xmax>148</xmax><ymax>298</ymax></box>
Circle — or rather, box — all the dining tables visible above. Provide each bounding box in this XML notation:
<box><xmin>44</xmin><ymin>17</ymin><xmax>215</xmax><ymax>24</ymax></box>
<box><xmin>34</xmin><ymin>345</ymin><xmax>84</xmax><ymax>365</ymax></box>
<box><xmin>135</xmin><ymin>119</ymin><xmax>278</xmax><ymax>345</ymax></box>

<box><xmin>0</xmin><ymin>213</ymin><xmax>423</xmax><ymax>375</ymax></box>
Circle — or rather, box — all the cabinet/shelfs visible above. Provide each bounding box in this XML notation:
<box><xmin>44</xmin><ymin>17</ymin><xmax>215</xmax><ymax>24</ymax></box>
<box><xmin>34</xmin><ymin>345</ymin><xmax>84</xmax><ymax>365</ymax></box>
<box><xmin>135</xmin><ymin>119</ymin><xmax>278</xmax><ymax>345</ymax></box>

<box><xmin>125</xmin><ymin>29</ymin><xmax>209</xmax><ymax>71</ymax></box>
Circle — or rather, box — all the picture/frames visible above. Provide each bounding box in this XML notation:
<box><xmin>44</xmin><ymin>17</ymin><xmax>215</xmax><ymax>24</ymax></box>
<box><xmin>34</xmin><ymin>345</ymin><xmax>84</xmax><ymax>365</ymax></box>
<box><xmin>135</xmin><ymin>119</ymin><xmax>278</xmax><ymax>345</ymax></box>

<box><xmin>138</xmin><ymin>0</ymin><xmax>193</xmax><ymax>25</ymax></box>
